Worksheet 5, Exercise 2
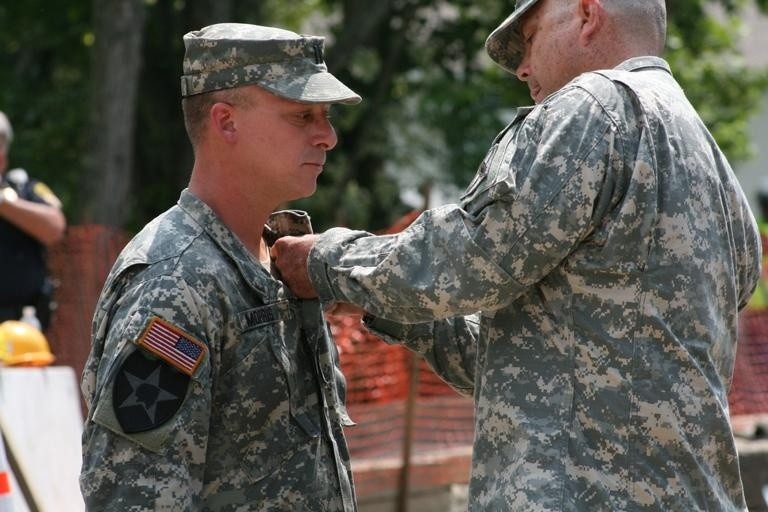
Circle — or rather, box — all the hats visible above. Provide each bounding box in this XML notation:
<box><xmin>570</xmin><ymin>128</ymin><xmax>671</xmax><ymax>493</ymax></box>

<box><xmin>486</xmin><ymin>0</ymin><xmax>538</xmax><ymax>74</ymax></box>
<box><xmin>181</xmin><ymin>22</ymin><xmax>362</xmax><ymax>106</ymax></box>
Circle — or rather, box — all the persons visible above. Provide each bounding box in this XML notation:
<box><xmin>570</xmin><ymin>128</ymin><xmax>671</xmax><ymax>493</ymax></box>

<box><xmin>0</xmin><ymin>110</ymin><xmax>69</xmax><ymax>340</ymax></box>
<box><xmin>77</xmin><ymin>20</ymin><xmax>372</xmax><ymax>512</ymax></box>
<box><xmin>268</xmin><ymin>1</ymin><xmax>765</xmax><ymax>512</ymax></box>
